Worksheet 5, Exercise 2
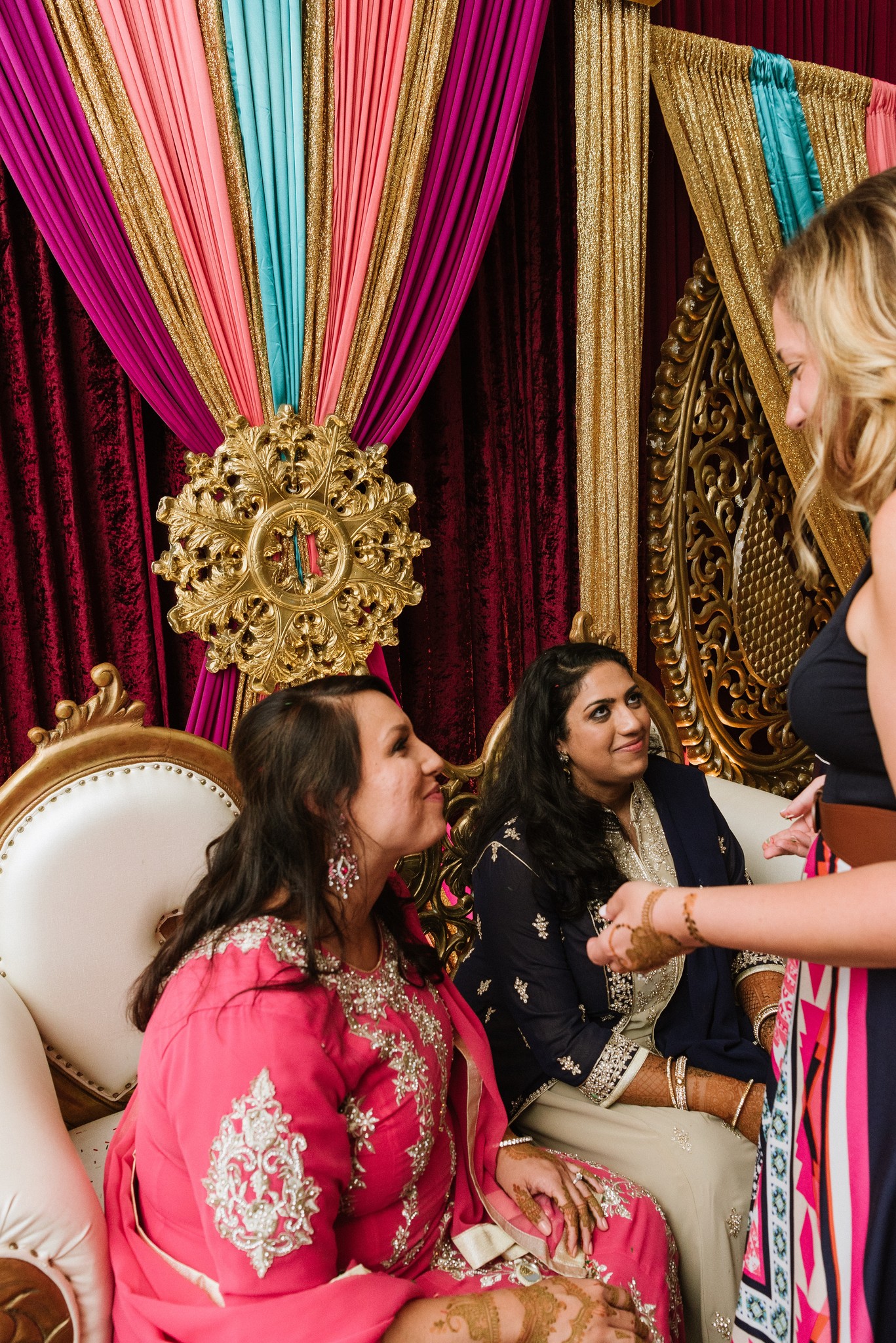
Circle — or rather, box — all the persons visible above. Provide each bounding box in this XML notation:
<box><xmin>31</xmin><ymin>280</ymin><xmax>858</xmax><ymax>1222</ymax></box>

<box><xmin>102</xmin><ymin>670</ymin><xmax>693</xmax><ymax>1343</ymax></box>
<box><xmin>441</xmin><ymin>650</ymin><xmax>792</xmax><ymax>1342</ymax></box>
<box><xmin>584</xmin><ymin>164</ymin><xmax>896</xmax><ymax>1343</ymax></box>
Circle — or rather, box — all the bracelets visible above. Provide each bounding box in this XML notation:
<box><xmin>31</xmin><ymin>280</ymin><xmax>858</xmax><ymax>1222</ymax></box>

<box><xmin>752</xmin><ymin>1003</ymin><xmax>780</xmax><ymax>1032</ymax></box>
<box><xmin>674</xmin><ymin>1056</ymin><xmax>690</xmax><ymax>1112</ymax></box>
<box><xmin>665</xmin><ymin>1055</ymin><xmax>678</xmax><ymax>1109</ymax></box>
<box><xmin>752</xmin><ymin>1006</ymin><xmax>780</xmax><ymax>1050</ymax></box>
<box><xmin>481</xmin><ymin>1135</ymin><xmax>535</xmax><ymax>1152</ymax></box>
<box><xmin>730</xmin><ymin>1078</ymin><xmax>755</xmax><ymax>1128</ymax></box>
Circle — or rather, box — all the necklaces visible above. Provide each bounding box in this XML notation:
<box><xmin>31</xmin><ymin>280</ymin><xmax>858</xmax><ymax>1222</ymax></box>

<box><xmin>624</xmin><ymin>820</ymin><xmax>633</xmax><ymax>838</ymax></box>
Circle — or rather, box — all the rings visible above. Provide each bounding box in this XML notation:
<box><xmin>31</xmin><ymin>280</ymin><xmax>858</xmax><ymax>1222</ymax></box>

<box><xmin>571</xmin><ymin>1171</ymin><xmax>585</xmax><ymax>1186</ymax></box>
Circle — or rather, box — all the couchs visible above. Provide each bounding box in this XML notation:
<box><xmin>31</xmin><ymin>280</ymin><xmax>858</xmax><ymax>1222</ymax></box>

<box><xmin>0</xmin><ymin>663</ymin><xmax>249</xmax><ymax>1342</ymax></box>
